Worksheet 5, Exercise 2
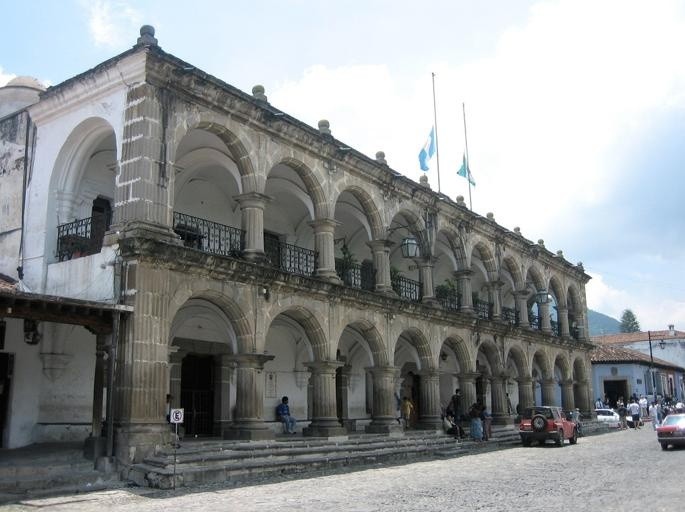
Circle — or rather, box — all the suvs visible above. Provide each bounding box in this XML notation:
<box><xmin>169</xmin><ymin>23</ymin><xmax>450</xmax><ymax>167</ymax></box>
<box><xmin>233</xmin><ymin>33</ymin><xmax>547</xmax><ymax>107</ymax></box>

<box><xmin>518</xmin><ymin>405</ymin><xmax>578</xmax><ymax>447</ymax></box>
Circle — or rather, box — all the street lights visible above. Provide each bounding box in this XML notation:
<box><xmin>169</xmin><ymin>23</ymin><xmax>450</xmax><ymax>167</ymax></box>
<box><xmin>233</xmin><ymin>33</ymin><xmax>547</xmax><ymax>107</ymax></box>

<box><xmin>648</xmin><ymin>331</ymin><xmax>666</xmax><ymax>404</ymax></box>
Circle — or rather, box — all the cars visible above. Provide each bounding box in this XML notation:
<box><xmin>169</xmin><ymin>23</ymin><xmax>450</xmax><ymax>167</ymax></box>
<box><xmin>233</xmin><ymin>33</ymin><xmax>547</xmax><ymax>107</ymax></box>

<box><xmin>655</xmin><ymin>414</ymin><xmax>684</xmax><ymax>450</ymax></box>
<box><xmin>595</xmin><ymin>408</ymin><xmax>644</xmax><ymax>429</ymax></box>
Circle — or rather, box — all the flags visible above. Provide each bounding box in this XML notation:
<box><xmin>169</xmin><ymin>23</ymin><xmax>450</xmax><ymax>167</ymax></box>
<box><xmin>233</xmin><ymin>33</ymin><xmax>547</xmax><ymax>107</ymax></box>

<box><xmin>455</xmin><ymin>152</ymin><xmax>475</xmax><ymax>185</ymax></box>
<box><xmin>417</xmin><ymin>124</ymin><xmax>436</xmax><ymax>173</ymax></box>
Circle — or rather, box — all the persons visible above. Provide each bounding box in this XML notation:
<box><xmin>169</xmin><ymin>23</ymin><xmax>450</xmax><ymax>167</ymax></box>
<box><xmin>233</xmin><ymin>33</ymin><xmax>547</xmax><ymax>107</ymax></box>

<box><xmin>506</xmin><ymin>392</ymin><xmax>514</xmax><ymax>416</ymax></box>
<box><xmin>167</xmin><ymin>394</ymin><xmax>182</xmax><ymax>440</ymax></box>
<box><xmin>276</xmin><ymin>395</ymin><xmax>297</xmax><ymax>434</ymax></box>
<box><xmin>571</xmin><ymin>390</ymin><xmax>685</xmax><ymax>439</ymax></box>
<box><xmin>394</xmin><ymin>388</ymin><xmax>494</xmax><ymax>443</ymax></box>
